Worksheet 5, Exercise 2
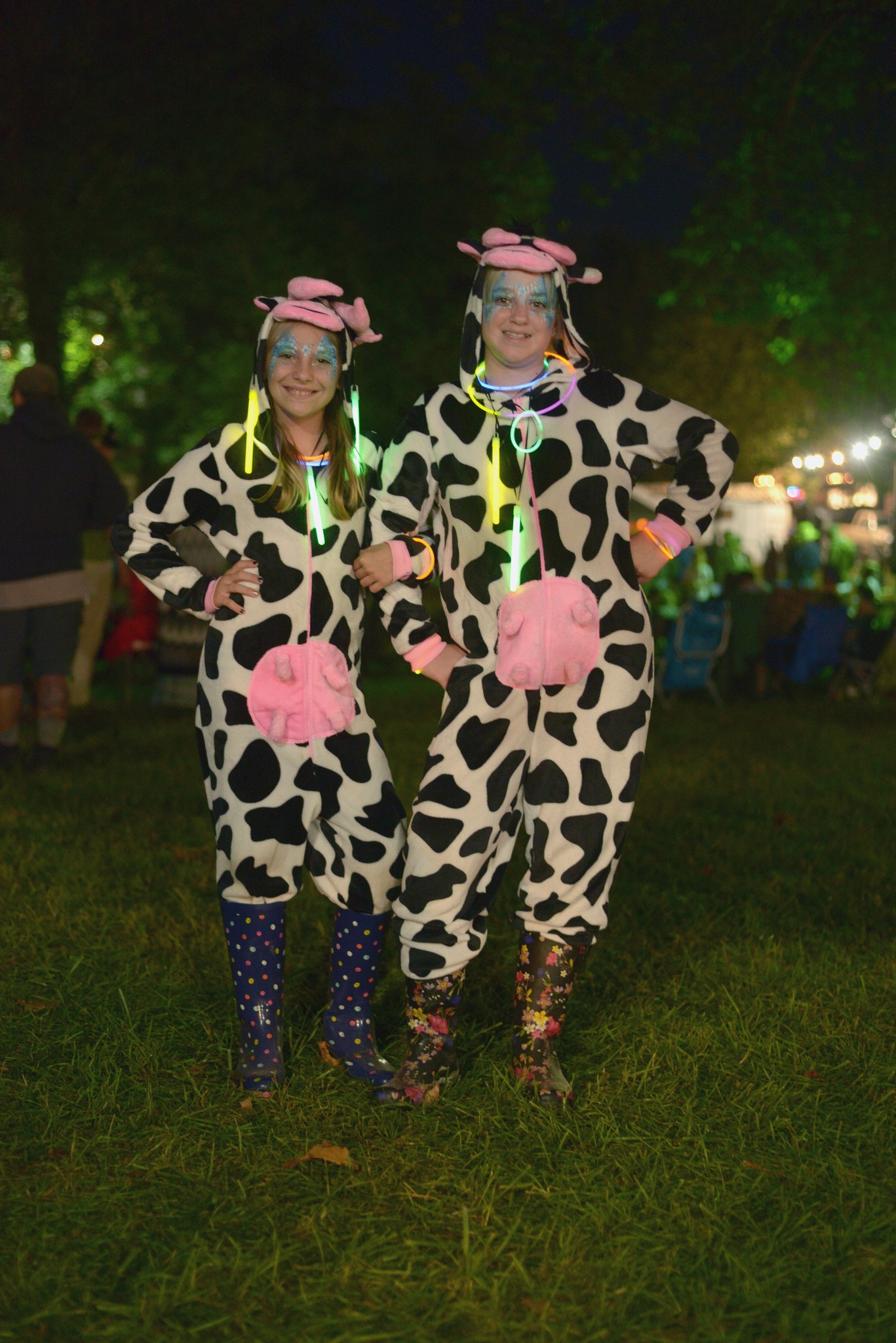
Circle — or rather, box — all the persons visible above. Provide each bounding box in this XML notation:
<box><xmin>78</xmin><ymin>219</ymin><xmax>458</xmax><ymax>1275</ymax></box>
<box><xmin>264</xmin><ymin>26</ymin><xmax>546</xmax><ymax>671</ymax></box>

<box><xmin>365</xmin><ymin>228</ymin><xmax>741</xmax><ymax>1105</ymax></box>
<box><xmin>648</xmin><ymin>502</ymin><xmax>895</xmax><ymax>704</ymax></box>
<box><xmin>111</xmin><ymin>276</ymin><xmax>436</xmax><ymax>1101</ymax></box>
<box><xmin>0</xmin><ymin>363</ymin><xmax>132</xmax><ymax>777</ymax></box>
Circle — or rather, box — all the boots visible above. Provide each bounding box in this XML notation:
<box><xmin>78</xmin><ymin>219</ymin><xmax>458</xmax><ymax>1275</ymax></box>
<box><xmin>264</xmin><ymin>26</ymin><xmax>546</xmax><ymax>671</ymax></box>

<box><xmin>219</xmin><ymin>899</ymin><xmax>289</xmax><ymax>1098</ymax></box>
<box><xmin>318</xmin><ymin>908</ymin><xmax>396</xmax><ymax>1087</ymax></box>
<box><xmin>373</xmin><ymin>967</ymin><xmax>464</xmax><ymax>1104</ymax></box>
<box><xmin>511</xmin><ymin>927</ymin><xmax>594</xmax><ymax>1110</ymax></box>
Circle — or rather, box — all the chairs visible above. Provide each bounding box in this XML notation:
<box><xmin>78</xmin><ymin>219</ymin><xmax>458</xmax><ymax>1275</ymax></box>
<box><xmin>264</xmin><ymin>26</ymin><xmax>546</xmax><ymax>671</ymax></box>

<box><xmin>155</xmin><ymin>525</ymin><xmax>225</xmax><ymax>668</ymax></box>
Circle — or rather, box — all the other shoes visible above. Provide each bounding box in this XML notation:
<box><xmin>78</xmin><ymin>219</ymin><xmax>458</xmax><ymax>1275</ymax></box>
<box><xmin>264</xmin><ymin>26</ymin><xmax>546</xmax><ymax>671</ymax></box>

<box><xmin>0</xmin><ymin>741</ymin><xmax>85</xmax><ymax>770</ymax></box>
<box><xmin>65</xmin><ymin>703</ymin><xmax>115</xmax><ymax>739</ymax></box>
